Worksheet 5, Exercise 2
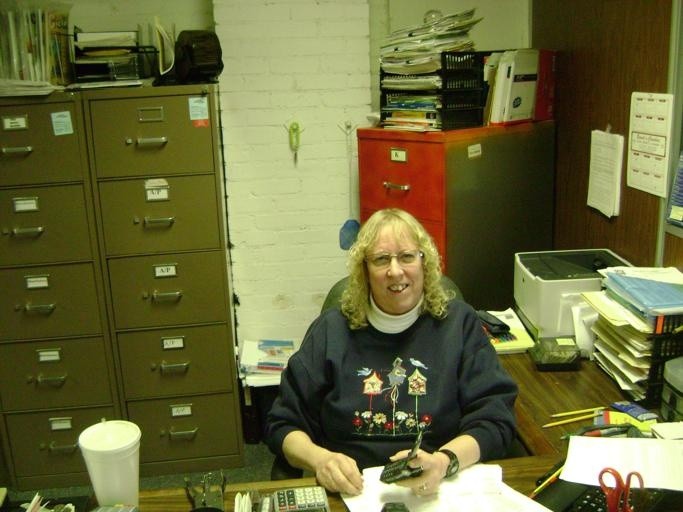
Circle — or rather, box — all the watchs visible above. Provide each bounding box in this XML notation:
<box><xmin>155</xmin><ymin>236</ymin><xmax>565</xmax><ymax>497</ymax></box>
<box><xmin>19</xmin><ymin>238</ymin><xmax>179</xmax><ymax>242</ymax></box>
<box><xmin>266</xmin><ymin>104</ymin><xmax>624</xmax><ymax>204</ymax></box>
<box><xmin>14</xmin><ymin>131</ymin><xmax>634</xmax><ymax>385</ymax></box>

<box><xmin>436</xmin><ymin>449</ymin><xmax>459</xmax><ymax>479</ymax></box>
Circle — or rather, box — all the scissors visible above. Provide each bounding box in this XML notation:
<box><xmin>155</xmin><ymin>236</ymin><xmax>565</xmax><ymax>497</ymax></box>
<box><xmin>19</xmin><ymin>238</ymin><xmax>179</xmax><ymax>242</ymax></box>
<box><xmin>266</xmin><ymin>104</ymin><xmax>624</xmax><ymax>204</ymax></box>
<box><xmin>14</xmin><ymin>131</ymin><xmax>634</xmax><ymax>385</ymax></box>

<box><xmin>599</xmin><ymin>467</ymin><xmax>643</xmax><ymax>512</ymax></box>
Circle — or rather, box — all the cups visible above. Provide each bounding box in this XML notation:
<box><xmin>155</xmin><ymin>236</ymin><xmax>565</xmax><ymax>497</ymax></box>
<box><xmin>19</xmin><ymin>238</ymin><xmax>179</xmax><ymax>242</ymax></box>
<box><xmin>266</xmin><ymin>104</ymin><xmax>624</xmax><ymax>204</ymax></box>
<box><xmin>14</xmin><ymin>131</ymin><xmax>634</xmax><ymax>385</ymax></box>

<box><xmin>79</xmin><ymin>420</ymin><xmax>141</xmax><ymax>508</ymax></box>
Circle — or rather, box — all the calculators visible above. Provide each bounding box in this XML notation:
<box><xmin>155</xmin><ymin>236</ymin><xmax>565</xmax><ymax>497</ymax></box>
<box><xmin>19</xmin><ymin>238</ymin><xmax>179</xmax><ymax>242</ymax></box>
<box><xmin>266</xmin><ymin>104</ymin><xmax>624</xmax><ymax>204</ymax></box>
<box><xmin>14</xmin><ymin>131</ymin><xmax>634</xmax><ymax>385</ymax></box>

<box><xmin>273</xmin><ymin>486</ymin><xmax>331</xmax><ymax>512</ymax></box>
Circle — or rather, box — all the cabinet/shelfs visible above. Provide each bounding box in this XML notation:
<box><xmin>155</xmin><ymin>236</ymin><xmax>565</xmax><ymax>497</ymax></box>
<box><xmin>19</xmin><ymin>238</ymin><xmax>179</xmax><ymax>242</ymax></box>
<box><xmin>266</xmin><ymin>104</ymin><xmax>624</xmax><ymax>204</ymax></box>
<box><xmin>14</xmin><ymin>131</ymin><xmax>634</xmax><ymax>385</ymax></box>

<box><xmin>380</xmin><ymin>50</ymin><xmax>491</xmax><ymax>132</ymax></box>
<box><xmin>0</xmin><ymin>83</ymin><xmax>245</xmax><ymax>491</ymax></box>
<box><xmin>595</xmin><ymin>315</ymin><xmax>683</xmax><ymax>409</ymax></box>
<box><xmin>357</xmin><ymin>120</ymin><xmax>556</xmax><ymax>309</ymax></box>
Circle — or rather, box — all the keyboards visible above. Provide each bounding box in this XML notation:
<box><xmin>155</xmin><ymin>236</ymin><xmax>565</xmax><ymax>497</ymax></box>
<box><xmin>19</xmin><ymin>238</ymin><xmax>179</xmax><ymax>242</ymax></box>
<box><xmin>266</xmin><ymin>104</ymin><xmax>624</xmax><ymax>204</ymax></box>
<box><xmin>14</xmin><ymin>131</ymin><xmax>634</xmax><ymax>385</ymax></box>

<box><xmin>572</xmin><ymin>487</ymin><xmax>665</xmax><ymax>512</ymax></box>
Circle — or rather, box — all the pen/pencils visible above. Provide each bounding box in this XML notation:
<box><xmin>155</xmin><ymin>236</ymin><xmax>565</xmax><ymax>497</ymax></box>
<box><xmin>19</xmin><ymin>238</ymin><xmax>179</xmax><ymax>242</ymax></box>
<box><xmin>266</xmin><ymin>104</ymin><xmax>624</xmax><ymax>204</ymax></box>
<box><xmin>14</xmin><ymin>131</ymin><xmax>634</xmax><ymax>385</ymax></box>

<box><xmin>529</xmin><ymin>459</ymin><xmax>566</xmax><ymax>499</ymax></box>
<box><xmin>541</xmin><ymin>406</ymin><xmax>628</xmax><ymax>440</ymax></box>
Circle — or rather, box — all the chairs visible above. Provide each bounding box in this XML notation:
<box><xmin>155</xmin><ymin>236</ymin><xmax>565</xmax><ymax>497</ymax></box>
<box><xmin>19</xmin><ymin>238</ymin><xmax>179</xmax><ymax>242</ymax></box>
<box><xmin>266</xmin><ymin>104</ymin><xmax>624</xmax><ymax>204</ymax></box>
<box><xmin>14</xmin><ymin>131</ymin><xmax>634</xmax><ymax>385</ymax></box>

<box><xmin>272</xmin><ymin>275</ymin><xmax>464</xmax><ymax>480</ymax></box>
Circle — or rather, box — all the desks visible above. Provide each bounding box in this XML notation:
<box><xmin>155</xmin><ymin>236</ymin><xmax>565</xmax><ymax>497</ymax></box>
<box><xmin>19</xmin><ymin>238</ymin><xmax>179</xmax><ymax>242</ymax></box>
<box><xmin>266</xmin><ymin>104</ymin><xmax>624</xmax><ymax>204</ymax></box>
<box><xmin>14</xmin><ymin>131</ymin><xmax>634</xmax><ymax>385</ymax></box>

<box><xmin>140</xmin><ymin>354</ymin><xmax>667</xmax><ymax>512</ymax></box>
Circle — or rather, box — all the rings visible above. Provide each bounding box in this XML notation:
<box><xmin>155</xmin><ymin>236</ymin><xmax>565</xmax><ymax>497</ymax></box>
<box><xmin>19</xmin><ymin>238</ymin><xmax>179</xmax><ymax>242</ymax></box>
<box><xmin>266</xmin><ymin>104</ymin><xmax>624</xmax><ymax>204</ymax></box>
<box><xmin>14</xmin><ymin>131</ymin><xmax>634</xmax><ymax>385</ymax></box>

<box><xmin>422</xmin><ymin>484</ymin><xmax>426</xmax><ymax>490</ymax></box>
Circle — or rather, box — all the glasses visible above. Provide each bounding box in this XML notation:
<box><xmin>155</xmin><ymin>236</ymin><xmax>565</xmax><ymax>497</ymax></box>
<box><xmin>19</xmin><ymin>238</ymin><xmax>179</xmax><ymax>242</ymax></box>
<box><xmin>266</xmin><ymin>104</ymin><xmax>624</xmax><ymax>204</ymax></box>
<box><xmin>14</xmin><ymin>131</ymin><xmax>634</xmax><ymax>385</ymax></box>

<box><xmin>363</xmin><ymin>250</ymin><xmax>423</xmax><ymax>266</ymax></box>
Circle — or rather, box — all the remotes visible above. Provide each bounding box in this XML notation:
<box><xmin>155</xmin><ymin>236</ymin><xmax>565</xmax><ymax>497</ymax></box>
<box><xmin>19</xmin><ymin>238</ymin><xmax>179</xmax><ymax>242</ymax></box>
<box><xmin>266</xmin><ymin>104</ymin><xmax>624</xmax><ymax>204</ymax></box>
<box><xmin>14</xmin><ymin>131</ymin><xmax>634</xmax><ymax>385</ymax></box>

<box><xmin>477</xmin><ymin>309</ymin><xmax>511</xmax><ymax>334</ymax></box>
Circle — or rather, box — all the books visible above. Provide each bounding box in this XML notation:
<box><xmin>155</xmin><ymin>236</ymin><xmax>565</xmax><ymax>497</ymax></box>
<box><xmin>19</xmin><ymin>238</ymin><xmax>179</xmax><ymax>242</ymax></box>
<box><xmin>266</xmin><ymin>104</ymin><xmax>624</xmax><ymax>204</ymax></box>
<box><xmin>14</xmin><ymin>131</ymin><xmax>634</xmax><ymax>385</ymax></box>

<box><xmin>0</xmin><ymin>5</ymin><xmax>65</xmax><ymax>98</ymax></box>
<box><xmin>571</xmin><ymin>264</ymin><xmax>683</xmax><ymax>402</ymax></box>
<box><xmin>381</xmin><ymin>9</ymin><xmax>483</xmax><ymax>134</ymax></box>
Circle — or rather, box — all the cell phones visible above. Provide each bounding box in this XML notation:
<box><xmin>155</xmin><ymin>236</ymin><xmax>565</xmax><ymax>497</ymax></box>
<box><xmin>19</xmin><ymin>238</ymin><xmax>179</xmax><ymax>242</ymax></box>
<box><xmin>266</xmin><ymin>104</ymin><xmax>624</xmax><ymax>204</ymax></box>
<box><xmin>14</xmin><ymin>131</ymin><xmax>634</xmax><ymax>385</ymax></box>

<box><xmin>380</xmin><ymin>430</ymin><xmax>423</xmax><ymax>484</ymax></box>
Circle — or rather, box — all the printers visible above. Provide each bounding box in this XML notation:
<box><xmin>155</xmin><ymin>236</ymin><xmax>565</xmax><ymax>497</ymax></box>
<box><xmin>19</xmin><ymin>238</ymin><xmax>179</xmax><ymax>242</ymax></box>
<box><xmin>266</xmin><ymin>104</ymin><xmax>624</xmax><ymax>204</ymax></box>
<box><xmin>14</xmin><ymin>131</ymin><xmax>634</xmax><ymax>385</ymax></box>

<box><xmin>512</xmin><ymin>247</ymin><xmax>636</xmax><ymax>341</ymax></box>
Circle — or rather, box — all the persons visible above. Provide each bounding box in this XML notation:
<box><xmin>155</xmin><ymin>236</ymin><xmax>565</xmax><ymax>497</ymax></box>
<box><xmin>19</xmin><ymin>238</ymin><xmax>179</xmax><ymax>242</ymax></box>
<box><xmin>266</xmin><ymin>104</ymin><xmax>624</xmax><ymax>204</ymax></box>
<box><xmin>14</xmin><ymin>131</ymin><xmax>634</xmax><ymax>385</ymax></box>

<box><xmin>261</xmin><ymin>209</ymin><xmax>520</xmax><ymax>498</ymax></box>
